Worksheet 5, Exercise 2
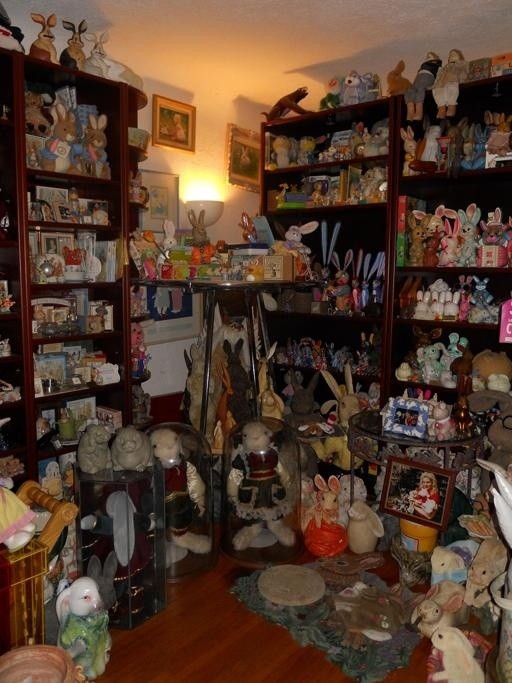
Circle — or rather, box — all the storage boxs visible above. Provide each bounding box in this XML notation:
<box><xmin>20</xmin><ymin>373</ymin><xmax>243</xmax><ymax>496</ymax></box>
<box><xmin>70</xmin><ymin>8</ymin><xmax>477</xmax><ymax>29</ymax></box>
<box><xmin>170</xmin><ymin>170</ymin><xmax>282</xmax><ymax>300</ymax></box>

<box><xmin>262</xmin><ymin>252</ymin><xmax>295</xmax><ymax>281</ymax></box>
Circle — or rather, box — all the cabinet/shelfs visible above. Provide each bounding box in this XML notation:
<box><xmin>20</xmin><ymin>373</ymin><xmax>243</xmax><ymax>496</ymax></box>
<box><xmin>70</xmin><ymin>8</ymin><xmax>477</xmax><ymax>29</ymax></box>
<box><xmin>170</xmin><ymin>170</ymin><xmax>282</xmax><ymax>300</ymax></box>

<box><xmin>250</xmin><ymin>96</ymin><xmax>400</xmax><ymax>496</ymax></box>
<box><xmin>1</xmin><ymin>46</ymin><xmax>38</xmax><ymax>496</ymax></box>
<box><xmin>384</xmin><ymin>70</ymin><xmax>512</xmax><ymax>565</ymax></box>
<box><xmin>17</xmin><ymin>52</ymin><xmax>150</xmax><ymax>510</ymax></box>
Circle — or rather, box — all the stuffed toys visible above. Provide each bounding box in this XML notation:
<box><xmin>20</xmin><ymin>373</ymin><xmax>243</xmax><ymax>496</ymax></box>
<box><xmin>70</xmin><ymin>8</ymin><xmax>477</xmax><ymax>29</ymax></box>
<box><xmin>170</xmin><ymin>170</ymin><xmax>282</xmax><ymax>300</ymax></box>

<box><xmin>0</xmin><ymin>0</ymin><xmax>512</xmax><ymax>681</ymax></box>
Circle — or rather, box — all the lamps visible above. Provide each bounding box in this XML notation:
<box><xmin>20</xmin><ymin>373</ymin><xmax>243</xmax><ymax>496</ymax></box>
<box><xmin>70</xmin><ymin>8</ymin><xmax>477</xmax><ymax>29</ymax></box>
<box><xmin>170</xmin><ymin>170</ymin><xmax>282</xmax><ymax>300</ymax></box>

<box><xmin>180</xmin><ymin>196</ymin><xmax>226</xmax><ymax>246</ymax></box>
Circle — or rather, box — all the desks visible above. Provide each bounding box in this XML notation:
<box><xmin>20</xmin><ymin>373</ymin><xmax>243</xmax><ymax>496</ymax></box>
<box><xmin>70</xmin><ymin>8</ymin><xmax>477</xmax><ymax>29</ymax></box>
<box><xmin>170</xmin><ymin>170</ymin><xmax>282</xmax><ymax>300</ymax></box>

<box><xmin>126</xmin><ymin>270</ymin><xmax>323</xmax><ymax>483</ymax></box>
<box><xmin>339</xmin><ymin>409</ymin><xmax>487</xmax><ymax>541</ymax></box>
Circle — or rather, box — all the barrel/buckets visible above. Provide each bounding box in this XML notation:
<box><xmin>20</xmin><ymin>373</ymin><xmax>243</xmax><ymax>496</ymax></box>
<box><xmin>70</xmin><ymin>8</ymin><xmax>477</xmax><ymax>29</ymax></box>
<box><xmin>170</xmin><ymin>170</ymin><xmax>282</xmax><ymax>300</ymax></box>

<box><xmin>399</xmin><ymin>517</ymin><xmax>438</xmax><ymax>554</ymax></box>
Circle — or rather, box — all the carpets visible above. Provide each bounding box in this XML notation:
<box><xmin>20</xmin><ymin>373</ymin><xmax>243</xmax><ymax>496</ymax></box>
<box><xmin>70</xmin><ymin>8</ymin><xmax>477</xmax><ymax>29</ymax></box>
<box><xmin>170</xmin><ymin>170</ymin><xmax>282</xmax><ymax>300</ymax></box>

<box><xmin>230</xmin><ymin>558</ymin><xmax>432</xmax><ymax>683</ymax></box>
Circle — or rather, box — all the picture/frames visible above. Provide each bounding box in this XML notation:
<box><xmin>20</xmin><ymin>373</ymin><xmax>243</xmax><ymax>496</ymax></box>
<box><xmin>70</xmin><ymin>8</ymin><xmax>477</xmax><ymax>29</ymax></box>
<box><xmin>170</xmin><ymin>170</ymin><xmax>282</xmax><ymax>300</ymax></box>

<box><xmin>135</xmin><ymin>167</ymin><xmax>182</xmax><ymax>237</ymax></box>
<box><xmin>130</xmin><ymin>282</ymin><xmax>205</xmax><ymax>348</ymax></box>
<box><xmin>382</xmin><ymin>396</ymin><xmax>431</xmax><ymax>440</ymax></box>
<box><xmin>148</xmin><ymin>90</ymin><xmax>199</xmax><ymax>155</ymax></box>
<box><xmin>225</xmin><ymin>120</ymin><xmax>264</xmax><ymax>197</ymax></box>
<box><xmin>378</xmin><ymin>455</ymin><xmax>458</xmax><ymax>533</ymax></box>
